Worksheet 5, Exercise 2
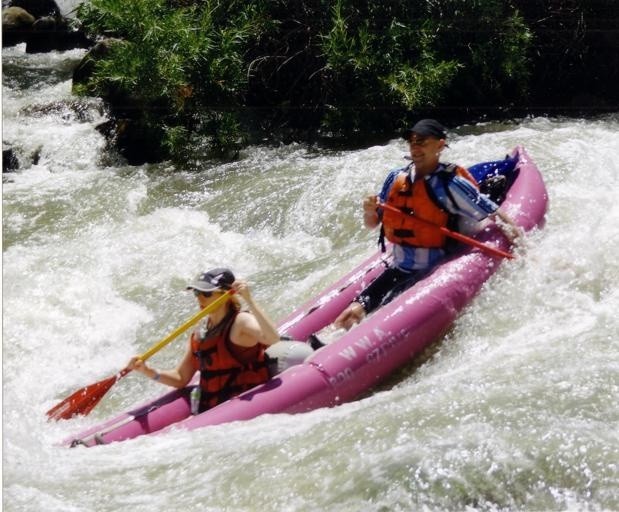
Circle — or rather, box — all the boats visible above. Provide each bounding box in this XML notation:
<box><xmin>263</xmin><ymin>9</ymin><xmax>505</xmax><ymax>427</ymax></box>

<box><xmin>53</xmin><ymin>145</ymin><xmax>549</xmax><ymax>448</ymax></box>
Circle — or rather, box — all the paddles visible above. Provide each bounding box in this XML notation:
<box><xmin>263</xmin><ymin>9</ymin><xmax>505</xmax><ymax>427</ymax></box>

<box><xmin>45</xmin><ymin>288</ymin><xmax>236</xmax><ymax>424</ymax></box>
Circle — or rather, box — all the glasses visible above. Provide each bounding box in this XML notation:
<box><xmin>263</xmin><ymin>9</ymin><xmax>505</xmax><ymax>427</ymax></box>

<box><xmin>405</xmin><ymin>139</ymin><xmax>426</xmax><ymax>146</ymax></box>
<box><xmin>192</xmin><ymin>288</ymin><xmax>213</xmax><ymax>298</ymax></box>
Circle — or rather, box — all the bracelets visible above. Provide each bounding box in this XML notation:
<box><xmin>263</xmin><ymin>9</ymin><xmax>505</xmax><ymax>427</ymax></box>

<box><xmin>153</xmin><ymin>369</ymin><xmax>160</xmax><ymax>381</ymax></box>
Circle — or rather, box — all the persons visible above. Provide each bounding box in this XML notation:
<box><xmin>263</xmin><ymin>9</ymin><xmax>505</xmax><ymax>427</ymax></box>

<box><xmin>331</xmin><ymin>118</ymin><xmax>525</xmax><ymax>333</ymax></box>
<box><xmin>129</xmin><ymin>267</ymin><xmax>280</xmax><ymax>417</ymax></box>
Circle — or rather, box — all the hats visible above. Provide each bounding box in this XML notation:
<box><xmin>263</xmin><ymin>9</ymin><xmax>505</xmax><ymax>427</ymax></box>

<box><xmin>184</xmin><ymin>267</ymin><xmax>235</xmax><ymax>292</ymax></box>
<box><xmin>401</xmin><ymin>119</ymin><xmax>448</xmax><ymax>140</ymax></box>
